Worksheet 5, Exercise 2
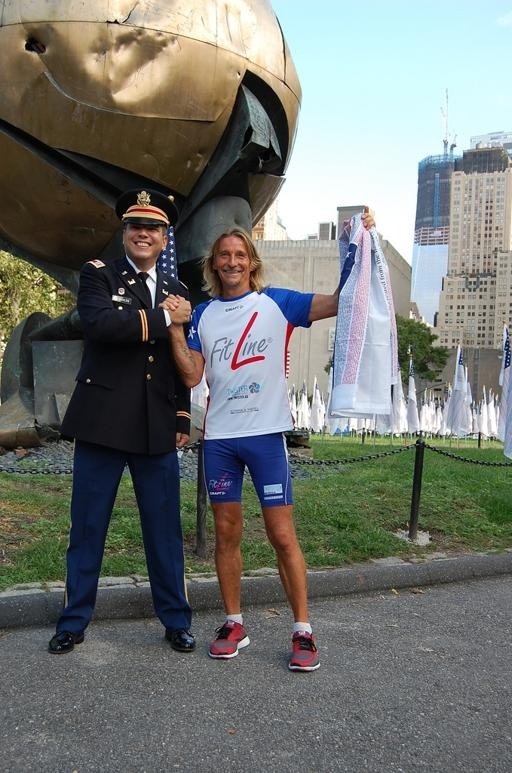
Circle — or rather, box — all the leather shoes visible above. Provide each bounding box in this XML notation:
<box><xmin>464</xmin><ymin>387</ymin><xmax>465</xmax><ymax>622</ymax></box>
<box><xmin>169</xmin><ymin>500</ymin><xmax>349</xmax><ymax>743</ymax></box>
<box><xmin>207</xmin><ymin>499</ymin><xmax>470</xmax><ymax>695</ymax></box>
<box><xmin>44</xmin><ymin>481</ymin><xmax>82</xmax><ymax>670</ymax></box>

<box><xmin>164</xmin><ymin>626</ymin><xmax>196</xmax><ymax>653</ymax></box>
<box><xmin>48</xmin><ymin>629</ymin><xmax>86</xmax><ymax>653</ymax></box>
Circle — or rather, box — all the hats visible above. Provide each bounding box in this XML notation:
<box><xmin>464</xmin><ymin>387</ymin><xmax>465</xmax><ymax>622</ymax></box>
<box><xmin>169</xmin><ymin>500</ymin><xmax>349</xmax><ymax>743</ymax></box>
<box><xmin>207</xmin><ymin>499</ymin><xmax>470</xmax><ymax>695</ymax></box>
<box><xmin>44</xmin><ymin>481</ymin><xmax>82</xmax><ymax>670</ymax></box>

<box><xmin>115</xmin><ymin>187</ymin><xmax>179</xmax><ymax>228</ymax></box>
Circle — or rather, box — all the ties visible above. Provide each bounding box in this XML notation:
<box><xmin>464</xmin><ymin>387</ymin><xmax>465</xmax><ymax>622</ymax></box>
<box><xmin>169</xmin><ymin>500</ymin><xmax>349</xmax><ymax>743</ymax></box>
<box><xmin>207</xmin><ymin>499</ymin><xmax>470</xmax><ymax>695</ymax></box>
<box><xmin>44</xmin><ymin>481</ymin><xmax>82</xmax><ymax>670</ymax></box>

<box><xmin>139</xmin><ymin>270</ymin><xmax>152</xmax><ymax>308</ymax></box>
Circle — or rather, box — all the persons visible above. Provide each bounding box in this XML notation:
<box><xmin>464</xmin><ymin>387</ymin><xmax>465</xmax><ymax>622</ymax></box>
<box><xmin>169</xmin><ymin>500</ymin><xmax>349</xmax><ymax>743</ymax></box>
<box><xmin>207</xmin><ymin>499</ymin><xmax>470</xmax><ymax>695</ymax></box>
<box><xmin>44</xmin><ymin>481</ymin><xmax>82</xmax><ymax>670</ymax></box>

<box><xmin>48</xmin><ymin>189</ymin><xmax>197</xmax><ymax>655</ymax></box>
<box><xmin>158</xmin><ymin>210</ymin><xmax>376</xmax><ymax>671</ymax></box>
<box><xmin>362</xmin><ymin>427</ymin><xmax>366</xmax><ymax>445</ymax></box>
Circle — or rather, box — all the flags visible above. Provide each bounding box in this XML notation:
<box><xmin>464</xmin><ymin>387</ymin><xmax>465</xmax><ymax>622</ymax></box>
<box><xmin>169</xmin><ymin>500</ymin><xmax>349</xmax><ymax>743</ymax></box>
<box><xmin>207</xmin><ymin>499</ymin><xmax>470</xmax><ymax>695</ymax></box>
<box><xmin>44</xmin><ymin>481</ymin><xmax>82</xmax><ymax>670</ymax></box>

<box><xmin>288</xmin><ymin>324</ymin><xmax>512</xmax><ymax>460</ymax></box>
<box><xmin>157</xmin><ymin>225</ymin><xmax>178</xmax><ymax>279</ymax></box>
<box><xmin>289</xmin><ymin>377</ymin><xmax>373</xmax><ymax>439</ymax></box>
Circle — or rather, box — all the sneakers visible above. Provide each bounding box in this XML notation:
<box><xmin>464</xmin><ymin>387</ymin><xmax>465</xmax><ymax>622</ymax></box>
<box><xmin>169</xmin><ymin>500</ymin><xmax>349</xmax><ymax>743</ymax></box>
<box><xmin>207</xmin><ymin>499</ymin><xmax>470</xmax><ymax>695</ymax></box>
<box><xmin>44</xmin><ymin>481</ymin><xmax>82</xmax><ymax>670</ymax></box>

<box><xmin>287</xmin><ymin>630</ymin><xmax>321</xmax><ymax>671</ymax></box>
<box><xmin>208</xmin><ymin>621</ymin><xmax>251</xmax><ymax>659</ymax></box>
